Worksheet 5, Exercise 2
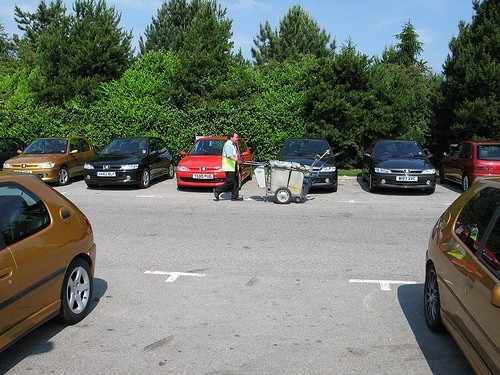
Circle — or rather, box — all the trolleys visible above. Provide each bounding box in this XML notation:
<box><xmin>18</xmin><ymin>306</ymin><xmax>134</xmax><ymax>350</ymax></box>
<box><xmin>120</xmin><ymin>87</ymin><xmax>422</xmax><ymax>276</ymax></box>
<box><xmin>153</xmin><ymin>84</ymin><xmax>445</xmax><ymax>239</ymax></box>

<box><xmin>239</xmin><ymin>158</ymin><xmax>318</xmax><ymax>204</ymax></box>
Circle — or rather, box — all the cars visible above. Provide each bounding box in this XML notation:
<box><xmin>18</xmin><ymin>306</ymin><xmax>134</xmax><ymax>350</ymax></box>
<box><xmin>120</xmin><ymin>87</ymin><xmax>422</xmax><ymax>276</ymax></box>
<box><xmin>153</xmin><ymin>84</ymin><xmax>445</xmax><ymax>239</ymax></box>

<box><xmin>84</xmin><ymin>137</ymin><xmax>175</xmax><ymax>189</ymax></box>
<box><xmin>176</xmin><ymin>136</ymin><xmax>252</xmax><ymax>189</ymax></box>
<box><xmin>439</xmin><ymin>140</ymin><xmax>500</xmax><ymax>192</ymax></box>
<box><xmin>0</xmin><ymin>171</ymin><xmax>96</xmax><ymax>354</ymax></box>
<box><xmin>0</xmin><ymin>137</ymin><xmax>100</xmax><ymax>187</ymax></box>
<box><xmin>361</xmin><ymin>140</ymin><xmax>435</xmax><ymax>194</ymax></box>
<box><xmin>270</xmin><ymin>139</ymin><xmax>344</xmax><ymax>192</ymax></box>
<box><xmin>423</xmin><ymin>177</ymin><xmax>499</xmax><ymax>375</ymax></box>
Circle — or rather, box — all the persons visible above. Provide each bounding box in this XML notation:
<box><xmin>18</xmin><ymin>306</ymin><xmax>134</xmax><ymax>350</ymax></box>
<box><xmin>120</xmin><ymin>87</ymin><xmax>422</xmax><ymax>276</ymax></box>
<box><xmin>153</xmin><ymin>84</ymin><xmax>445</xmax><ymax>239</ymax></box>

<box><xmin>455</xmin><ymin>218</ymin><xmax>478</xmax><ymax>250</ymax></box>
<box><xmin>213</xmin><ymin>131</ymin><xmax>243</xmax><ymax>201</ymax></box>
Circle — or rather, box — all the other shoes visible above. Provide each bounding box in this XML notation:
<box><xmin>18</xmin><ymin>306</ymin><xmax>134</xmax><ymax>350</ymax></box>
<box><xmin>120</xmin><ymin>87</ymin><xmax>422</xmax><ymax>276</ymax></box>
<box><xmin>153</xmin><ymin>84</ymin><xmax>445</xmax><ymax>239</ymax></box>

<box><xmin>231</xmin><ymin>196</ymin><xmax>243</xmax><ymax>201</ymax></box>
<box><xmin>213</xmin><ymin>188</ymin><xmax>220</xmax><ymax>201</ymax></box>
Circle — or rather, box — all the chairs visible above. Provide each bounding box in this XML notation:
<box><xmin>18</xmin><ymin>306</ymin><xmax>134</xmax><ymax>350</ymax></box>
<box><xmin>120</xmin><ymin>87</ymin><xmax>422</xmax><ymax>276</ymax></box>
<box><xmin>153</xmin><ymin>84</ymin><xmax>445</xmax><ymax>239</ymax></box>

<box><xmin>201</xmin><ymin>146</ymin><xmax>214</xmax><ymax>152</ymax></box>
<box><xmin>0</xmin><ymin>195</ymin><xmax>28</xmax><ymax>243</ymax></box>
